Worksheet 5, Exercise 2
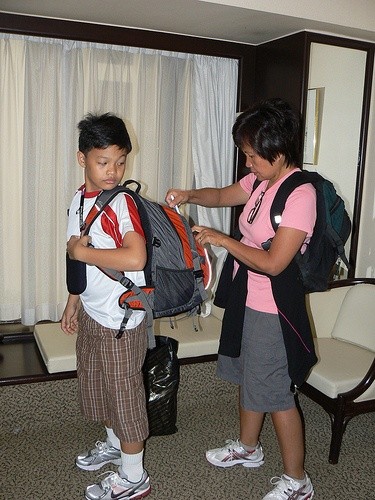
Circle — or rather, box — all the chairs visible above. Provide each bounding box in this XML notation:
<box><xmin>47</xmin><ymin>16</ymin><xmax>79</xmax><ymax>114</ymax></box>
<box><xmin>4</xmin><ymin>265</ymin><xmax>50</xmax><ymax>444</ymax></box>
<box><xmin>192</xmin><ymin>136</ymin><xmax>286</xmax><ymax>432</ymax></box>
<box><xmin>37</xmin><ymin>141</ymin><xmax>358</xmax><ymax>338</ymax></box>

<box><xmin>291</xmin><ymin>278</ymin><xmax>375</xmax><ymax>463</ymax></box>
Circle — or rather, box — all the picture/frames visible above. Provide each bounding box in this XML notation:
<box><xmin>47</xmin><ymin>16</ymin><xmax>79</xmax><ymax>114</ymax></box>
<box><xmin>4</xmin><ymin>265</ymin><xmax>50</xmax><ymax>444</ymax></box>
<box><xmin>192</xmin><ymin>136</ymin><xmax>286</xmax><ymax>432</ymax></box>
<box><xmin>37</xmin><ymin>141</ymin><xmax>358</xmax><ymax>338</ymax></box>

<box><xmin>304</xmin><ymin>89</ymin><xmax>320</xmax><ymax>165</ymax></box>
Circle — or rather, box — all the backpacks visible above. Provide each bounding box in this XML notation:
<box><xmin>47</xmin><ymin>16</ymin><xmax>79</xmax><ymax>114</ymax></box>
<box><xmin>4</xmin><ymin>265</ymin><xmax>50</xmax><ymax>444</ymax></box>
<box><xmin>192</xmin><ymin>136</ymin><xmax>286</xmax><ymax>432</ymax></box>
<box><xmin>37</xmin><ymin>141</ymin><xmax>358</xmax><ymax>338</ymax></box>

<box><xmin>248</xmin><ymin>164</ymin><xmax>350</xmax><ymax>292</ymax></box>
<box><xmin>79</xmin><ymin>179</ymin><xmax>214</xmax><ymax>319</ymax></box>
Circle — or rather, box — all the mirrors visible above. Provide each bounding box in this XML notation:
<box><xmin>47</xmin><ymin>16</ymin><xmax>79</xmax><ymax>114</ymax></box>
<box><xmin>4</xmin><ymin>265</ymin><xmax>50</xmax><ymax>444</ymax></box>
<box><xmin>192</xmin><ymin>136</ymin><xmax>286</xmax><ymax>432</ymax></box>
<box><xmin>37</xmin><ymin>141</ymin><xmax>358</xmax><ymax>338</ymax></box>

<box><xmin>305</xmin><ymin>43</ymin><xmax>375</xmax><ymax>283</ymax></box>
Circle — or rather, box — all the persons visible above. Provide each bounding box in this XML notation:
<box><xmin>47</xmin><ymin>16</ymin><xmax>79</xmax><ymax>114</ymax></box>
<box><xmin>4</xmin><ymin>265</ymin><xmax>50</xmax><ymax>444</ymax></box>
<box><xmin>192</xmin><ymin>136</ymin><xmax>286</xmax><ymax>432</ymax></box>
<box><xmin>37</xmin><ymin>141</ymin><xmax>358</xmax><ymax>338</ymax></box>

<box><xmin>164</xmin><ymin>96</ymin><xmax>317</xmax><ymax>500</ymax></box>
<box><xmin>61</xmin><ymin>111</ymin><xmax>150</xmax><ymax>500</ymax></box>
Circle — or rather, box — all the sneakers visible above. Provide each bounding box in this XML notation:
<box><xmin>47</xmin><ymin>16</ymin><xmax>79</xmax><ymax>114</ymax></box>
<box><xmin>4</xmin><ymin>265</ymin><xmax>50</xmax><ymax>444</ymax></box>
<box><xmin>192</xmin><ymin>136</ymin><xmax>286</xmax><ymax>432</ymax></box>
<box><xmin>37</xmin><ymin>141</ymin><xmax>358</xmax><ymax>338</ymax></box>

<box><xmin>206</xmin><ymin>439</ymin><xmax>265</xmax><ymax>468</ymax></box>
<box><xmin>262</xmin><ymin>471</ymin><xmax>314</xmax><ymax>500</ymax></box>
<box><xmin>84</xmin><ymin>465</ymin><xmax>151</xmax><ymax>500</ymax></box>
<box><xmin>75</xmin><ymin>441</ymin><xmax>123</xmax><ymax>471</ymax></box>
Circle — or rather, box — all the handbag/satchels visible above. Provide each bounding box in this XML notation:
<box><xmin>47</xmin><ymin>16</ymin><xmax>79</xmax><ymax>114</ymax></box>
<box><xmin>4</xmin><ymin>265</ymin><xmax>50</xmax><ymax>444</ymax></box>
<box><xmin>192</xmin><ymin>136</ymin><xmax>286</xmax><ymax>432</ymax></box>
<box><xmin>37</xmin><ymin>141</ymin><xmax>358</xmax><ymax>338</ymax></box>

<box><xmin>139</xmin><ymin>335</ymin><xmax>179</xmax><ymax>435</ymax></box>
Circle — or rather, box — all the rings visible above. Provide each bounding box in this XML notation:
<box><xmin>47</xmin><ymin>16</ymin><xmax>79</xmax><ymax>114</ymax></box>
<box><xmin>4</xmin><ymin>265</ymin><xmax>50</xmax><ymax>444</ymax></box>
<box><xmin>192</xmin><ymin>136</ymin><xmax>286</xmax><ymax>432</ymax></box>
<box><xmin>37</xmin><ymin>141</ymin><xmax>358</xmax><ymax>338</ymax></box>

<box><xmin>199</xmin><ymin>238</ymin><xmax>202</xmax><ymax>240</ymax></box>
